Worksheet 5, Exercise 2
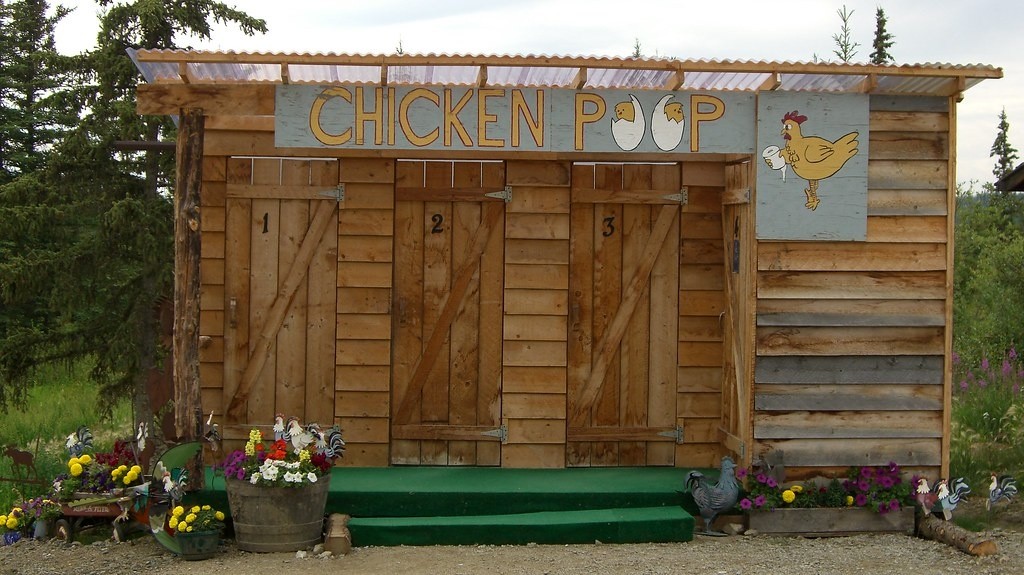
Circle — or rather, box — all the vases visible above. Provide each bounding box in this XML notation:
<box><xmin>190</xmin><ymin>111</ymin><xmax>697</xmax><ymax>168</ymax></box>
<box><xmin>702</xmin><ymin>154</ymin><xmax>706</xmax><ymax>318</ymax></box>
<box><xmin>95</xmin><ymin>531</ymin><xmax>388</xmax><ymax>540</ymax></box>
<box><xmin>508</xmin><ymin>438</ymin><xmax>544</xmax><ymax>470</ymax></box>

<box><xmin>63</xmin><ymin>492</ymin><xmax>131</xmax><ymax>517</ymax></box>
<box><xmin>749</xmin><ymin>506</ymin><xmax>916</xmax><ymax>538</ymax></box>
<box><xmin>225</xmin><ymin>474</ymin><xmax>332</xmax><ymax>553</ymax></box>
<box><xmin>176</xmin><ymin>529</ymin><xmax>221</xmax><ymax>561</ymax></box>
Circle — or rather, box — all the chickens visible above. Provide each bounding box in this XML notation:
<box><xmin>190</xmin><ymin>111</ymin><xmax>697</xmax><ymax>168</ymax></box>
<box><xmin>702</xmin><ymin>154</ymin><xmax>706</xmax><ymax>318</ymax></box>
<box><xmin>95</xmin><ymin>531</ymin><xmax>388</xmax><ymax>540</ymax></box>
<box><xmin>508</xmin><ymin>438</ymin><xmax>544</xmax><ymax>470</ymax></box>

<box><xmin>685</xmin><ymin>456</ymin><xmax>738</xmax><ymax>537</ymax></box>
<box><xmin>162</xmin><ymin>468</ymin><xmax>188</xmax><ymax>504</ymax></box>
<box><xmin>916</xmin><ymin>475</ymin><xmax>971</xmax><ymax>520</ymax></box>
<box><xmin>272</xmin><ymin>413</ymin><xmax>346</xmax><ymax>461</ymax></box>
<box><xmin>989</xmin><ymin>473</ymin><xmax>1017</xmax><ymax>508</ymax></box>
<box><xmin>65</xmin><ymin>424</ymin><xmax>94</xmax><ymax>459</ymax></box>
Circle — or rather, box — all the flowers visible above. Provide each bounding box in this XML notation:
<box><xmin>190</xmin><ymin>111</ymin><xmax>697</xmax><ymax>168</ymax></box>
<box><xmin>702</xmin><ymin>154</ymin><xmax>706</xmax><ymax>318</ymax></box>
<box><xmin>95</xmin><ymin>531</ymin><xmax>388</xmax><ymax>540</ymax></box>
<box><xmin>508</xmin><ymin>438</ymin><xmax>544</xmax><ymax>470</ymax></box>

<box><xmin>213</xmin><ymin>428</ymin><xmax>336</xmax><ymax>490</ymax></box>
<box><xmin>736</xmin><ymin>460</ymin><xmax>921</xmax><ymax>514</ymax></box>
<box><xmin>169</xmin><ymin>505</ymin><xmax>225</xmax><ymax>533</ymax></box>
<box><xmin>0</xmin><ymin>437</ymin><xmax>143</xmax><ymax>542</ymax></box>
